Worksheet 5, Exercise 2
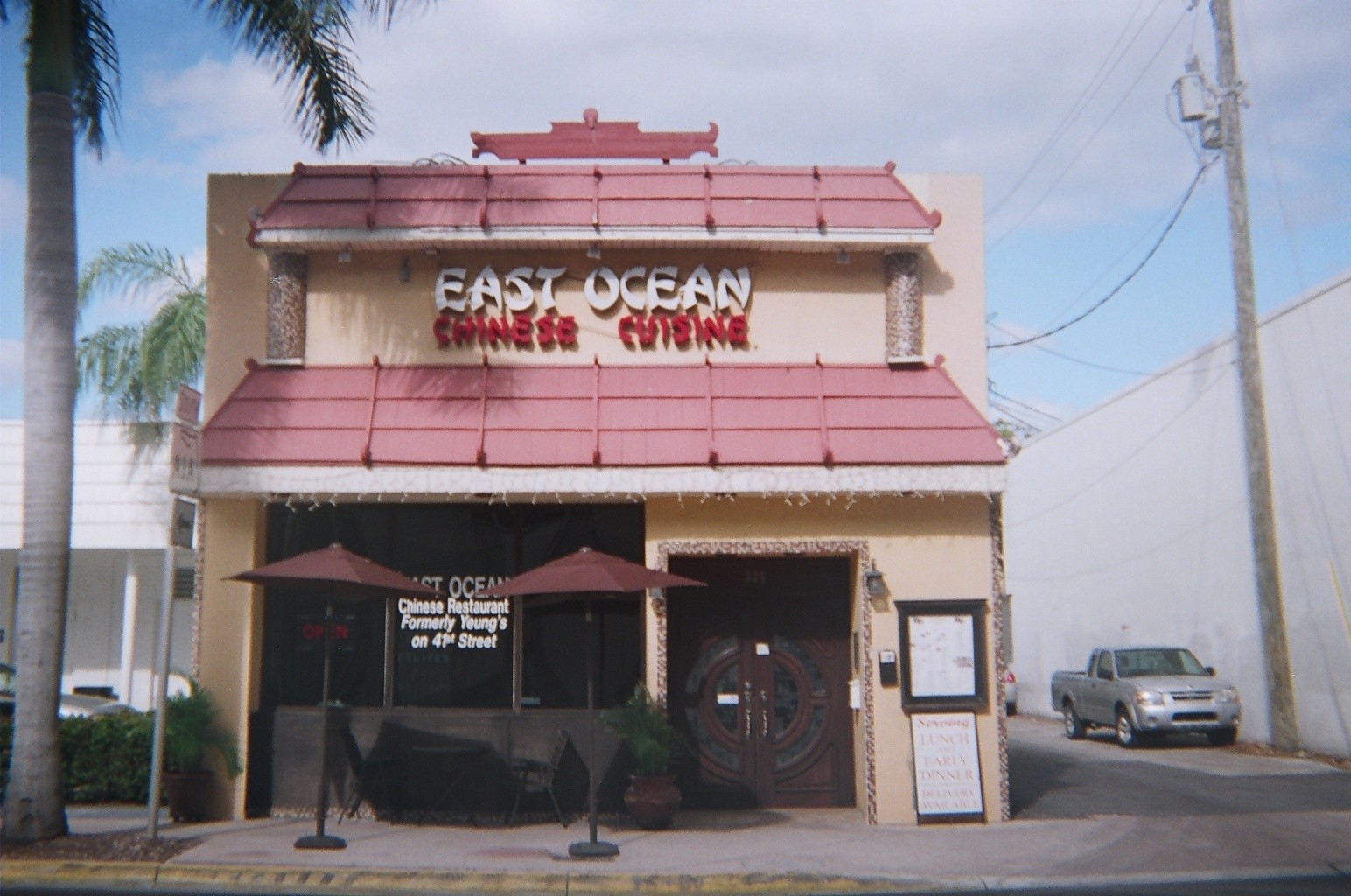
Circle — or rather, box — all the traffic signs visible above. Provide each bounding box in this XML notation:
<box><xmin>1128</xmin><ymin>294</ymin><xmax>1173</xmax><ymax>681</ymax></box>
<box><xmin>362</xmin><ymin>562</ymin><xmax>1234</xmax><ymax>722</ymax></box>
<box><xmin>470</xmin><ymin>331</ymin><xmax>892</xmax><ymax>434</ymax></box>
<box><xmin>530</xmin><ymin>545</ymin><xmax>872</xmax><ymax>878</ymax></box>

<box><xmin>170</xmin><ymin>500</ymin><xmax>197</xmax><ymax>549</ymax></box>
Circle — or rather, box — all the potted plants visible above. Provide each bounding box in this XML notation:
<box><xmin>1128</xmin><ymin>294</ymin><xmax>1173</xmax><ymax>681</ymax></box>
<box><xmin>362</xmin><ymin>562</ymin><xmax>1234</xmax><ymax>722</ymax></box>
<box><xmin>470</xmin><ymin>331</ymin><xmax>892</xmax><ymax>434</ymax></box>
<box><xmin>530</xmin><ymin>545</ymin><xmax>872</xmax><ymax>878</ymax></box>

<box><xmin>600</xmin><ymin>680</ymin><xmax>690</xmax><ymax>823</ymax></box>
<box><xmin>148</xmin><ymin>674</ymin><xmax>243</xmax><ymax>823</ymax></box>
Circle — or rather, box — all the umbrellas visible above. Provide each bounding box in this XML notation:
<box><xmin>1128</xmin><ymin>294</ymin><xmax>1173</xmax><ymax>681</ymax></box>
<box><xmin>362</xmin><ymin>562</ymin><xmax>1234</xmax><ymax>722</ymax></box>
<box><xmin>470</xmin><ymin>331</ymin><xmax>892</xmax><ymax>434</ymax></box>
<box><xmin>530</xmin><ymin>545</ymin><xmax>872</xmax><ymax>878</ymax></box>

<box><xmin>219</xmin><ymin>542</ymin><xmax>451</xmax><ymax>850</ymax></box>
<box><xmin>473</xmin><ymin>546</ymin><xmax>710</xmax><ymax>858</ymax></box>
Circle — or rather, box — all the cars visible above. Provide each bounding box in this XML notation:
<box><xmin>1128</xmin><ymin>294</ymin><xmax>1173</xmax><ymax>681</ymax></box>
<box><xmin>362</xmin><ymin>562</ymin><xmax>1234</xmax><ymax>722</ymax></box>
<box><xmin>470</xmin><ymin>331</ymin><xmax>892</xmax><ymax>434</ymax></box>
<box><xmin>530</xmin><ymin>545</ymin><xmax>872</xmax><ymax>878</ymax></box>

<box><xmin>1003</xmin><ymin>665</ymin><xmax>1016</xmax><ymax>715</ymax></box>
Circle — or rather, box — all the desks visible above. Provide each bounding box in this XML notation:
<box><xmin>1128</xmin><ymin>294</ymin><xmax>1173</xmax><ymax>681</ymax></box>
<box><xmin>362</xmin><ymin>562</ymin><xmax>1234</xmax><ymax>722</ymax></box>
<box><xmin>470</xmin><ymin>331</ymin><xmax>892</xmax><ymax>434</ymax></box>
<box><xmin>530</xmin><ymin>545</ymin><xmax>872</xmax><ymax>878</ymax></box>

<box><xmin>411</xmin><ymin>744</ymin><xmax>485</xmax><ymax>822</ymax></box>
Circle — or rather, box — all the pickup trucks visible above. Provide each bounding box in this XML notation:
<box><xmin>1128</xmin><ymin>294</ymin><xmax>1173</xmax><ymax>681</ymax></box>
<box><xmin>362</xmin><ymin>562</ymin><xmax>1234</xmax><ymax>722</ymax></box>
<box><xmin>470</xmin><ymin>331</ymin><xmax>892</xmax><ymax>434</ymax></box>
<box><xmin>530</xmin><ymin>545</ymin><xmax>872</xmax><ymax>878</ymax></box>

<box><xmin>1051</xmin><ymin>643</ymin><xmax>1241</xmax><ymax>747</ymax></box>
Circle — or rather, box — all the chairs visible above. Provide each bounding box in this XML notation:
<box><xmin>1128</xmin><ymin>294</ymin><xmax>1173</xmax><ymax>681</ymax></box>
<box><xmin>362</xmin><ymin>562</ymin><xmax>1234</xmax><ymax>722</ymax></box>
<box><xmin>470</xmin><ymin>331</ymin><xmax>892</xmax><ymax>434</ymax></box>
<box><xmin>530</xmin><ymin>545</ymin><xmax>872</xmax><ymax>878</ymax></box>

<box><xmin>336</xmin><ymin>725</ymin><xmax>403</xmax><ymax>826</ymax></box>
<box><xmin>507</xmin><ymin>726</ymin><xmax>572</xmax><ymax>828</ymax></box>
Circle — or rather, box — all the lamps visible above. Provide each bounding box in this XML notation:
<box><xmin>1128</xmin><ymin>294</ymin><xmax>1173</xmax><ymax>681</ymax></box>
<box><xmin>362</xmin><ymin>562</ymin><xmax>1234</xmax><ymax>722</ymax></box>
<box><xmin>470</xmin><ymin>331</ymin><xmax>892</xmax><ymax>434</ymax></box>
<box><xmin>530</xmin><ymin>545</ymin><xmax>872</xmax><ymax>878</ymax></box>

<box><xmin>865</xmin><ymin>559</ymin><xmax>886</xmax><ymax>595</ymax></box>
